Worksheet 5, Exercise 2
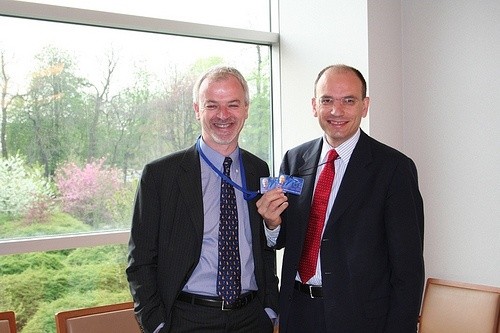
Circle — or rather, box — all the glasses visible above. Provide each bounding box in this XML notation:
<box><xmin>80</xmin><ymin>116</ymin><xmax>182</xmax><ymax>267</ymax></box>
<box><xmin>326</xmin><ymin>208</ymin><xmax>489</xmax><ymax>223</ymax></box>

<box><xmin>316</xmin><ymin>95</ymin><xmax>363</xmax><ymax>110</ymax></box>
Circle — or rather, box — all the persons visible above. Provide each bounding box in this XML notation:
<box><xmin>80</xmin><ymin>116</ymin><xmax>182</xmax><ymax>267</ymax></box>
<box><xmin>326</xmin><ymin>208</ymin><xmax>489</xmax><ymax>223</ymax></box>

<box><xmin>256</xmin><ymin>64</ymin><xmax>425</xmax><ymax>333</ymax></box>
<box><xmin>125</xmin><ymin>67</ymin><xmax>279</xmax><ymax>333</ymax></box>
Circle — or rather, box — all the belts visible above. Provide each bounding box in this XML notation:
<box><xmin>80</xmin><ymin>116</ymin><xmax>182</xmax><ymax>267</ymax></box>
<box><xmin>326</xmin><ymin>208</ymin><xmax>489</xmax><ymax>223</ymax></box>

<box><xmin>295</xmin><ymin>279</ymin><xmax>324</xmax><ymax>298</ymax></box>
<box><xmin>178</xmin><ymin>291</ymin><xmax>257</xmax><ymax>310</ymax></box>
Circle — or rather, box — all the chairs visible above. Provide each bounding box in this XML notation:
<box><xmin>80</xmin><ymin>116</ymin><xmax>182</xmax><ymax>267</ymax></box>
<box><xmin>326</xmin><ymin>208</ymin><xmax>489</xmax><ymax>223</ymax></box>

<box><xmin>0</xmin><ymin>311</ymin><xmax>16</xmax><ymax>333</ymax></box>
<box><xmin>417</xmin><ymin>278</ymin><xmax>500</xmax><ymax>333</ymax></box>
<box><xmin>55</xmin><ymin>301</ymin><xmax>141</xmax><ymax>332</ymax></box>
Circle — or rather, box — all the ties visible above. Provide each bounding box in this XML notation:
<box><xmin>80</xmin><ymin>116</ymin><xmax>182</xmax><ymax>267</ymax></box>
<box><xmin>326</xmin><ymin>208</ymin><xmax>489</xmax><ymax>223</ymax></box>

<box><xmin>216</xmin><ymin>157</ymin><xmax>241</xmax><ymax>307</ymax></box>
<box><xmin>298</xmin><ymin>150</ymin><xmax>338</xmax><ymax>283</ymax></box>
